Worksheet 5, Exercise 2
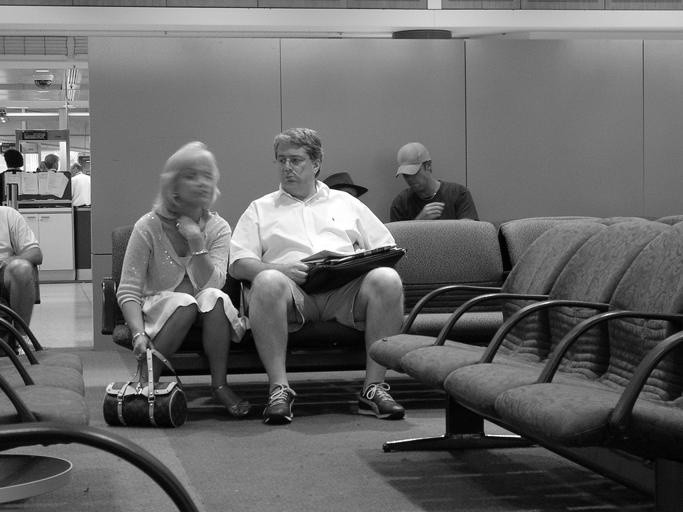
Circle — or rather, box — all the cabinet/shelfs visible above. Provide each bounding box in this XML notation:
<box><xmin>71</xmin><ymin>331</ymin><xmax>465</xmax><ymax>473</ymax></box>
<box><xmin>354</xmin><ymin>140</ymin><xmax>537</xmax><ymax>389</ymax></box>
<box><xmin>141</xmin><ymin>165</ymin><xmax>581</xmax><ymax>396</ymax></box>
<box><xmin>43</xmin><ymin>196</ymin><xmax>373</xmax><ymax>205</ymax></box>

<box><xmin>3</xmin><ymin>199</ymin><xmax>75</xmax><ymax>283</ymax></box>
<box><xmin>75</xmin><ymin>206</ymin><xmax>93</xmax><ymax>282</ymax></box>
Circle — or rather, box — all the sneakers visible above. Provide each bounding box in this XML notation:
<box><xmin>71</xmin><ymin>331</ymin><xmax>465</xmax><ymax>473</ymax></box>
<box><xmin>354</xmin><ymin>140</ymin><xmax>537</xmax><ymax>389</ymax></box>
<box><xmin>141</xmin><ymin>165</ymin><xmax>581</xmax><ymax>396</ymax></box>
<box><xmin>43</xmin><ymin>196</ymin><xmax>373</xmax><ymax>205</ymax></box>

<box><xmin>262</xmin><ymin>385</ymin><xmax>297</xmax><ymax>425</ymax></box>
<box><xmin>358</xmin><ymin>381</ymin><xmax>406</xmax><ymax>420</ymax></box>
<box><xmin>10</xmin><ymin>333</ymin><xmax>29</xmax><ymax>356</ymax></box>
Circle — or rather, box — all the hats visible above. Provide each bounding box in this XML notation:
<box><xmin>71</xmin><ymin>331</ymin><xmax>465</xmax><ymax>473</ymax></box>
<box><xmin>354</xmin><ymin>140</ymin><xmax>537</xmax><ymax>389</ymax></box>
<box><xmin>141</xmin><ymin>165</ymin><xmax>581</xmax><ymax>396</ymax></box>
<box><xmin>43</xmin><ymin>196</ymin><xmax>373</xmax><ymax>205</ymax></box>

<box><xmin>322</xmin><ymin>172</ymin><xmax>368</xmax><ymax>197</ymax></box>
<box><xmin>395</xmin><ymin>141</ymin><xmax>431</xmax><ymax>175</ymax></box>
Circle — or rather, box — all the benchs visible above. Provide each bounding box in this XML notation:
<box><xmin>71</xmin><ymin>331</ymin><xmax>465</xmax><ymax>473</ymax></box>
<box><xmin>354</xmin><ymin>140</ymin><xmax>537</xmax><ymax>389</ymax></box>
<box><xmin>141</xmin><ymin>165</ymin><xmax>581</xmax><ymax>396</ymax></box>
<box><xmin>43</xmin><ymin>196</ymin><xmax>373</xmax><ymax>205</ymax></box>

<box><xmin>371</xmin><ymin>221</ymin><xmax>683</xmax><ymax>511</ymax></box>
<box><xmin>0</xmin><ymin>304</ymin><xmax>89</xmax><ymax>444</ymax></box>
<box><xmin>0</xmin><ymin>423</ymin><xmax>199</xmax><ymax>512</ymax></box>
<box><xmin>100</xmin><ymin>216</ymin><xmax>599</xmax><ymax>383</ymax></box>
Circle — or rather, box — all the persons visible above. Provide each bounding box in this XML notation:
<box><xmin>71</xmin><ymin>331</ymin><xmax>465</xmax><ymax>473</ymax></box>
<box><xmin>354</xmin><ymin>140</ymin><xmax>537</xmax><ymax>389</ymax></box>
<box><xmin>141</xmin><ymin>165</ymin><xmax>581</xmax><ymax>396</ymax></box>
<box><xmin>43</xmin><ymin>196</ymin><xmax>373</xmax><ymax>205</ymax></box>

<box><xmin>35</xmin><ymin>154</ymin><xmax>59</xmax><ymax>178</ymax></box>
<box><xmin>116</xmin><ymin>141</ymin><xmax>251</xmax><ymax>418</ymax></box>
<box><xmin>1</xmin><ymin>150</ymin><xmax>25</xmax><ymax>195</ymax></box>
<box><xmin>390</xmin><ymin>142</ymin><xmax>480</xmax><ymax>222</ymax></box>
<box><xmin>0</xmin><ymin>206</ymin><xmax>43</xmax><ymax>353</ymax></box>
<box><xmin>229</xmin><ymin>128</ymin><xmax>405</xmax><ymax>425</ymax></box>
<box><xmin>70</xmin><ymin>150</ymin><xmax>91</xmax><ymax>208</ymax></box>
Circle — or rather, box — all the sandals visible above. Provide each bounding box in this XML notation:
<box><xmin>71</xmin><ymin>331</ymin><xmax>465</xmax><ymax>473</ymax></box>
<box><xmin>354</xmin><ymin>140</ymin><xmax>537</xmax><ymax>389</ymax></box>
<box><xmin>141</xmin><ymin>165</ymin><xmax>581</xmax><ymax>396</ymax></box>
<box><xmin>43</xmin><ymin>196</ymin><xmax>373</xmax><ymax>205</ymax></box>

<box><xmin>211</xmin><ymin>382</ymin><xmax>254</xmax><ymax>420</ymax></box>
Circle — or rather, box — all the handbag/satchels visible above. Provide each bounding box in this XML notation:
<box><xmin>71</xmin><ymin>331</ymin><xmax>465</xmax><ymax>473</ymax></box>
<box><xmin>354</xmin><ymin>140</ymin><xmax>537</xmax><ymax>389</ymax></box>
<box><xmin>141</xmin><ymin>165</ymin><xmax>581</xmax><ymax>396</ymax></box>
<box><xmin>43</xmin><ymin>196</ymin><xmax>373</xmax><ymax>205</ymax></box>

<box><xmin>301</xmin><ymin>245</ymin><xmax>410</xmax><ymax>297</ymax></box>
<box><xmin>103</xmin><ymin>347</ymin><xmax>189</xmax><ymax>428</ymax></box>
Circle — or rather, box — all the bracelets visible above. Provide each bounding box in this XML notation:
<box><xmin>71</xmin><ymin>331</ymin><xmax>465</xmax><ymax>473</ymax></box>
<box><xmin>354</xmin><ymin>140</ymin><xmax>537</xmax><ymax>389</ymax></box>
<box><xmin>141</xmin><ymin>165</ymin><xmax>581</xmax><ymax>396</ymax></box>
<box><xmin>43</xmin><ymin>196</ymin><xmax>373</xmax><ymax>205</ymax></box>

<box><xmin>131</xmin><ymin>332</ymin><xmax>146</xmax><ymax>344</ymax></box>
<box><xmin>192</xmin><ymin>249</ymin><xmax>209</xmax><ymax>255</ymax></box>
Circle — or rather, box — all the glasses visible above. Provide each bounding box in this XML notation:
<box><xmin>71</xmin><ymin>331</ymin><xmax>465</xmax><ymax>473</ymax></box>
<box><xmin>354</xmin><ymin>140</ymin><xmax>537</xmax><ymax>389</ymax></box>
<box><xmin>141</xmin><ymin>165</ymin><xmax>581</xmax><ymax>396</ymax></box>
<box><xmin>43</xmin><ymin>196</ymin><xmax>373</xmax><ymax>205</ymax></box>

<box><xmin>272</xmin><ymin>157</ymin><xmax>315</xmax><ymax>166</ymax></box>
